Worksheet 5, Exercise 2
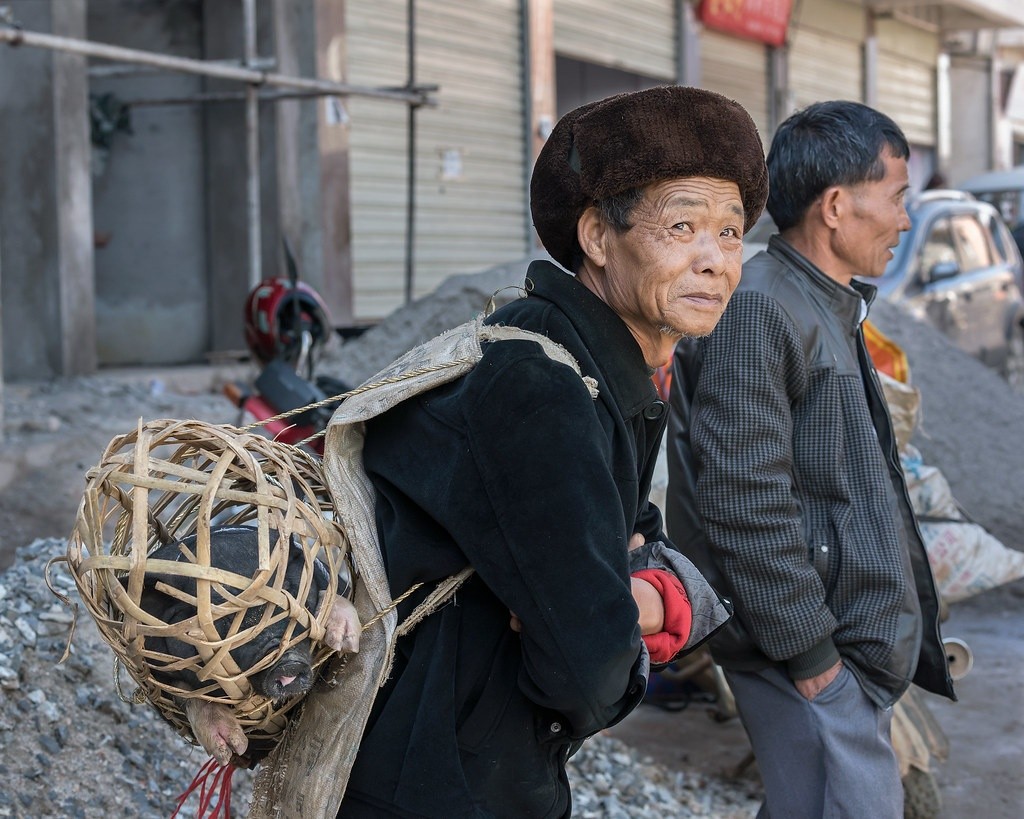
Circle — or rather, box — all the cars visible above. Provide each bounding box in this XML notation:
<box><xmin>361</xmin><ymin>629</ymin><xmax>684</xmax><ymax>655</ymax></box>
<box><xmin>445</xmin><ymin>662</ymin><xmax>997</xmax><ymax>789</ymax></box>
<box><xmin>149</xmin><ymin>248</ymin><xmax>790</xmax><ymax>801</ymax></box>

<box><xmin>962</xmin><ymin>164</ymin><xmax>1024</xmax><ymax>239</ymax></box>
<box><xmin>739</xmin><ymin>188</ymin><xmax>1024</xmax><ymax>389</ymax></box>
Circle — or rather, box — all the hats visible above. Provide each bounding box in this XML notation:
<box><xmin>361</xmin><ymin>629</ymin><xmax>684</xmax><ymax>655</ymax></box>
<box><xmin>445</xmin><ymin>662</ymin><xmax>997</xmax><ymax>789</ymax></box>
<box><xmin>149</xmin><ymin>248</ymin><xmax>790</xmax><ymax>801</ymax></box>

<box><xmin>529</xmin><ymin>86</ymin><xmax>772</xmax><ymax>275</ymax></box>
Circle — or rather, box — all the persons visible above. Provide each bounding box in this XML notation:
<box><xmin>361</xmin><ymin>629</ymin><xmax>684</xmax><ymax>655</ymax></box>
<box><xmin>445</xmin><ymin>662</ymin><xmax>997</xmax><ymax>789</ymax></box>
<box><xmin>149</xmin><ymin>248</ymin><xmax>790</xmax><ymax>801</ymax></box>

<box><xmin>669</xmin><ymin>100</ymin><xmax>959</xmax><ymax>819</ymax></box>
<box><xmin>236</xmin><ymin>87</ymin><xmax>768</xmax><ymax>819</ymax></box>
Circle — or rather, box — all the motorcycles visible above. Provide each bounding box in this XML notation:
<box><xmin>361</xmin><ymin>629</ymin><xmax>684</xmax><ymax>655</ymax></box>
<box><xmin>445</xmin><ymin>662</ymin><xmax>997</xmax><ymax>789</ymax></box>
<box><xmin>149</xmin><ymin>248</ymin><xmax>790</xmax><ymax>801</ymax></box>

<box><xmin>222</xmin><ymin>232</ymin><xmax>975</xmax><ymax>818</ymax></box>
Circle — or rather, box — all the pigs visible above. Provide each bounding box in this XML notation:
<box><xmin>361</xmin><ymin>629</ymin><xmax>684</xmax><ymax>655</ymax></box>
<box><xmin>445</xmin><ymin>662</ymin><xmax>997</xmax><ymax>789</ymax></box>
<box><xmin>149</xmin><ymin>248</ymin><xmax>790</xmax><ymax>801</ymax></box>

<box><xmin>109</xmin><ymin>525</ymin><xmax>362</xmax><ymax>764</ymax></box>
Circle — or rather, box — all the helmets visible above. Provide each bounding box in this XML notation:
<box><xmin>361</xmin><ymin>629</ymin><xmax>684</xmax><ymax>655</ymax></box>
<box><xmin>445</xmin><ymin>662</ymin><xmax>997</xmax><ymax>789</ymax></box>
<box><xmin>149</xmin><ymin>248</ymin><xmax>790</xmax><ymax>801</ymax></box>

<box><xmin>241</xmin><ymin>275</ymin><xmax>335</xmax><ymax>368</ymax></box>
